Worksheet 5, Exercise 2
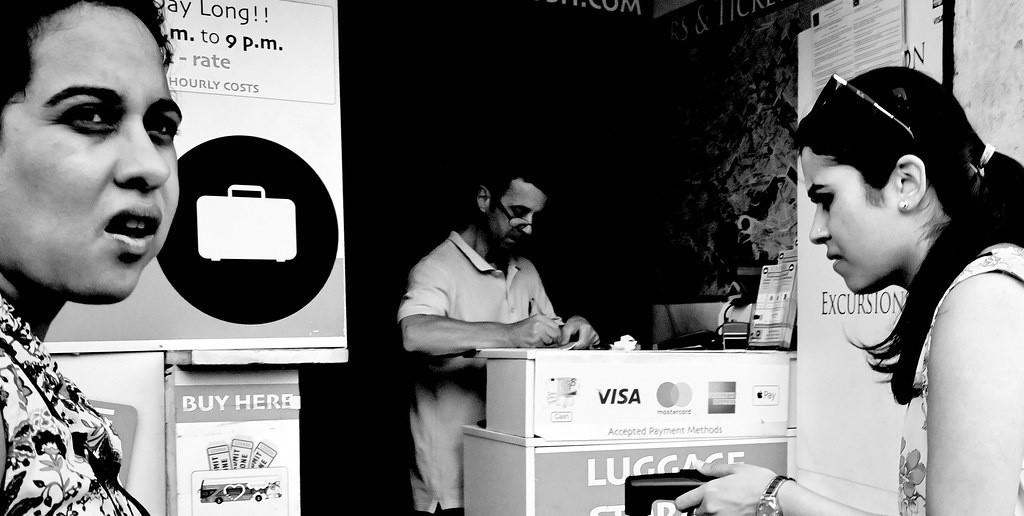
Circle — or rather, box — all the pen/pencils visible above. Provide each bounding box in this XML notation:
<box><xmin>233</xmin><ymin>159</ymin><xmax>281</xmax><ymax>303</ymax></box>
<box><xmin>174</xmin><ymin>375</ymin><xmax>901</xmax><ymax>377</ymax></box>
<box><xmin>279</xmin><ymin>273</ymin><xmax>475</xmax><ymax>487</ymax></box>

<box><xmin>531</xmin><ymin>298</ymin><xmax>562</xmax><ymax>347</ymax></box>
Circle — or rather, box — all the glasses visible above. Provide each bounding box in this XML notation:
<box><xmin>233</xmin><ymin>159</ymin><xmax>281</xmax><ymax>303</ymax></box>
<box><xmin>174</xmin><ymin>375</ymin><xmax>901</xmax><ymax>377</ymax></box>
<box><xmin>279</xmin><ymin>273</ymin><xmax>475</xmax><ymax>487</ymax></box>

<box><xmin>491</xmin><ymin>191</ymin><xmax>533</xmax><ymax>230</ymax></box>
<box><xmin>811</xmin><ymin>73</ymin><xmax>915</xmax><ymax>139</ymax></box>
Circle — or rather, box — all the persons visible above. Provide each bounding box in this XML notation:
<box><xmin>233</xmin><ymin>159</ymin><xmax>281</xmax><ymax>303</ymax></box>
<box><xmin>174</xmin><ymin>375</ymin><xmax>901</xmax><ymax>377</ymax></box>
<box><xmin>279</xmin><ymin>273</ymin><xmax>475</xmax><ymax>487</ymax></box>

<box><xmin>398</xmin><ymin>150</ymin><xmax>601</xmax><ymax>516</ymax></box>
<box><xmin>673</xmin><ymin>66</ymin><xmax>1024</xmax><ymax>516</ymax></box>
<box><xmin>0</xmin><ymin>0</ymin><xmax>182</xmax><ymax>516</ymax></box>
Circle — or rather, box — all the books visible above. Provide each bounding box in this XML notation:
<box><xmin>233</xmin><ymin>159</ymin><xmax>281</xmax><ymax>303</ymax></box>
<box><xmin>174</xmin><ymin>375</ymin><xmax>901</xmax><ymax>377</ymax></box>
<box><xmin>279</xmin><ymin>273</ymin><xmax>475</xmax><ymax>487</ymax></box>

<box><xmin>749</xmin><ymin>246</ymin><xmax>798</xmax><ymax>348</ymax></box>
<box><xmin>475</xmin><ymin>341</ymin><xmax>578</xmax><ymax>350</ymax></box>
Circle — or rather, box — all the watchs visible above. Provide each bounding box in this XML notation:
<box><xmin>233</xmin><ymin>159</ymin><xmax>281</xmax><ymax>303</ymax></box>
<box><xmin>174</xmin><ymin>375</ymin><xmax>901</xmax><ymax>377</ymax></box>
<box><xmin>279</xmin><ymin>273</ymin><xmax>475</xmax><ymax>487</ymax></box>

<box><xmin>754</xmin><ymin>475</ymin><xmax>797</xmax><ymax>516</ymax></box>
